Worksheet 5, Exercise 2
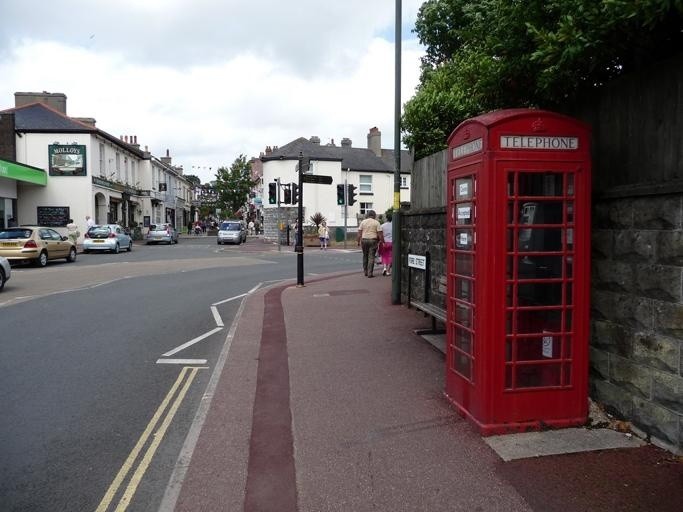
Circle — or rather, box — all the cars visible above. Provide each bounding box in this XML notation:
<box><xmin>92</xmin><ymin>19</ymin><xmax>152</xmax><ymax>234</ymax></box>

<box><xmin>82</xmin><ymin>224</ymin><xmax>133</xmax><ymax>254</ymax></box>
<box><xmin>0</xmin><ymin>226</ymin><xmax>77</xmax><ymax>268</ymax></box>
<box><xmin>216</xmin><ymin>220</ymin><xmax>247</xmax><ymax>245</ymax></box>
<box><xmin>146</xmin><ymin>222</ymin><xmax>179</xmax><ymax>245</ymax></box>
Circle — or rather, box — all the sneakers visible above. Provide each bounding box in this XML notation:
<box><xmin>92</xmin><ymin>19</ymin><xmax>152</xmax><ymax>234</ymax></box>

<box><xmin>381</xmin><ymin>268</ymin><xmax>392</xmax><ymax>276</ymax></box>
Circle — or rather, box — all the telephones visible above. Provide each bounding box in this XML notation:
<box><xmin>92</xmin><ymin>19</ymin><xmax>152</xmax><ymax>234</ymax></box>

<box><xmin>514</xmin><ymin>202</ymin><xmax>545</xmax><ymax>266</ymax></box>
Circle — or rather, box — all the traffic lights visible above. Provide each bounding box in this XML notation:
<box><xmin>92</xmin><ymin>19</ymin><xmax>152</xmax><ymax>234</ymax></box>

<box><xmin>269</xmin><ymin>182</ymin><xmax>276</xmax><ymax>204</ymax></box>
<box><xmin>293</xmin><ymin>182</ymin><xmax>300</xmax><ymax>205</ymax></box>
<box><xmin>337</xmin><ymin>184</ymin><xmax>344</xmax><ymax>206</ymax></box>
<box><xmin>348</xmin><ymin>184</ymin><xmax>358</xmax><ymax>206</ymax></box>
<box><xmin>284</xmin><ymin>189</ymin><xmax>291</xmax><ymax>205</ymax></box>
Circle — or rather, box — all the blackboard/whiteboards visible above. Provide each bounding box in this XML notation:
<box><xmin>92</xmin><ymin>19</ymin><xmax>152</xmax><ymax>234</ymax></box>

<box><xmin>37</xmin><ymin>206</ymin><xmax>70</xmax><ymax>227</ymax></box>
<box><xmin>144</xmin><ymin>216</ymin><xmax>150</xmax><ymax>227</ymax></box>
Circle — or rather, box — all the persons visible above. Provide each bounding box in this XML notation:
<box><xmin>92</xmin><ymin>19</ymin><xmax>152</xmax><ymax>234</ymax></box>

<box><xmin>317</xmin><ymin>222</ymin><xmax>329</xmax><ymax>250</ymax></box>
<box><xmin>248</xmin><ymin>220</ymin><xmax>254</xmax><ymax>236</ymax></box>
<box><xmin>85</xmin><ymin>216</ymin><xmax>92</xmax><ymax>229</ymax></box>
<box><xmin>65</xmin><ymin>219</ymin><xmax>77</xmax><ymax>249</ymax></box>
<box><xmin>187</xmin><ymin>216</ymin><xmax>219</xmax><ymax>237</ymax></box>
<box><xmin>292</xmin><ymin>217</ymin><xmax>304</xmax><ymax>251</ymax></box>
<box><xmin>379</xmin><ymin>213</ymin><xmax>392</xmax><ymax>276</ymax></box>
<box><xmin>377</xmin><ymin>214</ymin><xmax>384</xmax><ymax>225</ymax></box>
<box><xmin>356</xmin><ymin>209</ymin><xmax>384</xmax><ymax>278</ymax></box>
<box><xmin>253</xmin><ymin>220</ymin><xmax>259</xmax><ymax>235</ymax></box>
